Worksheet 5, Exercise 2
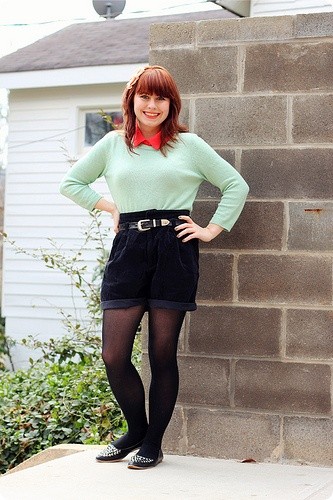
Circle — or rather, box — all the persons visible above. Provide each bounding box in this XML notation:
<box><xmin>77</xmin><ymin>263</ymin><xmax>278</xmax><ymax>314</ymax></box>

<box><xmin>61</xmin><ymin>64</ymin><xmax>249</xmax><ymax>470</ymax></box>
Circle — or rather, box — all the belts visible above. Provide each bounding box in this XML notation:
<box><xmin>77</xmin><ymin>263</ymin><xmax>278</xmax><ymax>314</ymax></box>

<box><xmin>118</xmin><ymin>219</ymin><xmax>187</xmax><ymax>232</ymax></box>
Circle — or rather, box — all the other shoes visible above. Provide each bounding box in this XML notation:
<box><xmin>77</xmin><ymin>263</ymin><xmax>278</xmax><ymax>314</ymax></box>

<box><xmin>95</xmin><ymin>441</ymin><xmax>145</xmax><ymax>463</ymax></box>
<box><xmin>127</xmin><ymin>449</ymin><xmax>163</xmax><ymax>470</ymax></box>
